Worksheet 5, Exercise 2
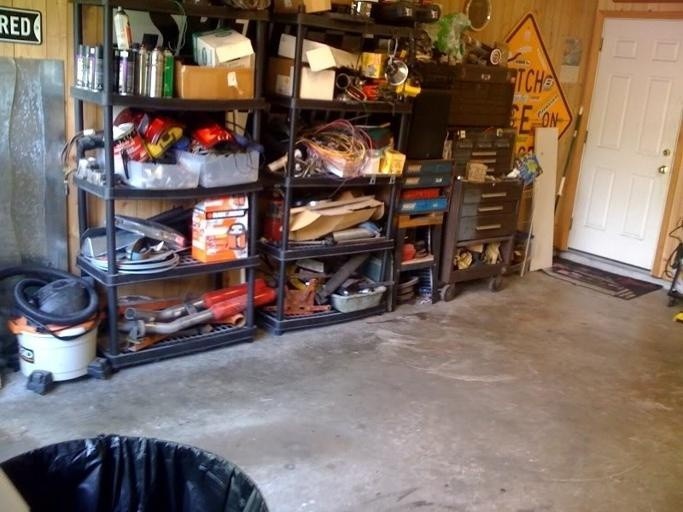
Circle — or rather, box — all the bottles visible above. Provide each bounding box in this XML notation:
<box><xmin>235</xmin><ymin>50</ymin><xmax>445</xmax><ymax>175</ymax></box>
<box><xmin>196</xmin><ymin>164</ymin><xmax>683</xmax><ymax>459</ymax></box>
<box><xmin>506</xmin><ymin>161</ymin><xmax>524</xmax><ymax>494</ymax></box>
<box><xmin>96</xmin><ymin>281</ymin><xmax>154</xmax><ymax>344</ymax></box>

<box><xmin>114</xmin><ymin>5</ymin><xmax>134</xmax><ymax>48</ymax></box>
<box><xmin>134</xmin><ymin>33</ymin><xmax>177</xmax><ymax>99</ymax></box>
<box><xmin>75</xmin><ymin>44</ymin><xmax>139</xmax><ymax>96</ymax></box>
<box><xmin>402</xmin><ymin>243</ymin><xmax>416</xmax><ymax>261</ymax></box>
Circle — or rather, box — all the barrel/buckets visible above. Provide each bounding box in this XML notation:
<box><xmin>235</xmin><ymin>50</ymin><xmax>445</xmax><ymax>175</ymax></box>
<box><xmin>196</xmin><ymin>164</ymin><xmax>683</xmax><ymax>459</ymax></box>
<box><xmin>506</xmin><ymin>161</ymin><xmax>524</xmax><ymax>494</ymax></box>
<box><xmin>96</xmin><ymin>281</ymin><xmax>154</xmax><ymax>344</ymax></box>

<box><xmin>101</xmin><ymin>123</ymin><xmax>154</xmax><ymax>163</ymax></box>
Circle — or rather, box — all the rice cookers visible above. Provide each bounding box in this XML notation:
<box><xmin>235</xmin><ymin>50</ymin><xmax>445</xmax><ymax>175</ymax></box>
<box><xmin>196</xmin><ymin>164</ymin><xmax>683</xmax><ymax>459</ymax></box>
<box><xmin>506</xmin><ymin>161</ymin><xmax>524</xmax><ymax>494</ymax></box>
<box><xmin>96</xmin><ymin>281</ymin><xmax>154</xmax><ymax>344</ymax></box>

<box><xmin>227</xmin><ymin>223</ymin><xmax>247</xmax><ymax>250</ymax></box>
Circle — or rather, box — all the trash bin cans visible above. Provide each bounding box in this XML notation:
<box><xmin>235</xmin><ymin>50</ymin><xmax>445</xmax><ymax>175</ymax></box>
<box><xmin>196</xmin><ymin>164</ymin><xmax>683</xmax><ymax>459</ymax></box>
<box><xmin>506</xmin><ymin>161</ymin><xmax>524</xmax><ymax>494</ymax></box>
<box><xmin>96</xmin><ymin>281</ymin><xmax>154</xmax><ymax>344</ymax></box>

<box><xmin>0</xmin><ymin>433</ymin><xmax>270</xmax><ymax>512</ymax></box>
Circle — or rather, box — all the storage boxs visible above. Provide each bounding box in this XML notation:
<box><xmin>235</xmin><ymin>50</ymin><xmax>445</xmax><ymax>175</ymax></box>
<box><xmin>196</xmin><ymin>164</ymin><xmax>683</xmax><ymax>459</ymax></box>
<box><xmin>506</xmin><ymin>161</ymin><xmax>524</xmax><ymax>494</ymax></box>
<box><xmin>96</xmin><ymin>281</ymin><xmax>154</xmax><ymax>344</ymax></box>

<box><xmin>268</xmin><ymin>54</ymin><xmax>335</xmax><ymax>100</ymax></box>
<box><xmin>175</xmin><ymin>59</ymin><xmax>255</xmax><ymax>100</ymax></box>
<box><xmin>172</xmin><ymin>146</ymin><xmax>259</xmax><ymax>190</ymax></box>
<box><xmin>113</xmin><ymin>155</ymin><xmax>202</xmax><ymax>193</ymax></box>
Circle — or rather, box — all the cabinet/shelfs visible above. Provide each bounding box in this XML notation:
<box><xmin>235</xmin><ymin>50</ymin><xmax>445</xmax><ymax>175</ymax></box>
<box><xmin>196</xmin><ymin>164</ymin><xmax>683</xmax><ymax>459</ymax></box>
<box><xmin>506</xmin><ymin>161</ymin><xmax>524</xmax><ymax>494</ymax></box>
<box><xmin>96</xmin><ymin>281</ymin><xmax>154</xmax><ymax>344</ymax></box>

<box><xmin>69</xmin><ymin>0</ymin><xmax>271</xmax><ymax>369</ymax></box>
<box><xmin>255</xmin><ymin>5</ymin><xmax>421</xmax><ymax>335</ymax></box>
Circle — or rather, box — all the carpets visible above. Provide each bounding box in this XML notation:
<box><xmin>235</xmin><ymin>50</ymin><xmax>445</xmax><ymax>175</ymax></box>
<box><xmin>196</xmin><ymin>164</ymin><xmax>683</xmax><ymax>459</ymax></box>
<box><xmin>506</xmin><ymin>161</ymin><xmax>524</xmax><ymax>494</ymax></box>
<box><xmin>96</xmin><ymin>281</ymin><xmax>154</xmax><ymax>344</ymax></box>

<box><xmin>539</xmin><ymin>255</ymin><xmax>662</xmax><ymax>301</ymax></box>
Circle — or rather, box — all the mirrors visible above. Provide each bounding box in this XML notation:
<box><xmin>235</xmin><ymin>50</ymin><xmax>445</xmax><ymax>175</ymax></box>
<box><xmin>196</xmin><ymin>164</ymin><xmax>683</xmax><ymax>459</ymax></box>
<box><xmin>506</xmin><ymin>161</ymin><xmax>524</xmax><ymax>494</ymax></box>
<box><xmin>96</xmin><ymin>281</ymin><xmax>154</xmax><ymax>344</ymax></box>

<box><xmin>464</xmin><ymin>0</ymin><xmax>492</xmax><ymax>31</ymax></box>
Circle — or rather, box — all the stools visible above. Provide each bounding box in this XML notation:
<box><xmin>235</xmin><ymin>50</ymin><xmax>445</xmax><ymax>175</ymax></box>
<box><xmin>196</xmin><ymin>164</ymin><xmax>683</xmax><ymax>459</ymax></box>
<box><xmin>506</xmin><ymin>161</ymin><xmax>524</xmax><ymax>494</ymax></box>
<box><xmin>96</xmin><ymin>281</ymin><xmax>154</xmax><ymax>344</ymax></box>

<box><xmin>392</xmin><ymin>211</ymin><xmax>445</xmax><ymax>306</ymax></box>
